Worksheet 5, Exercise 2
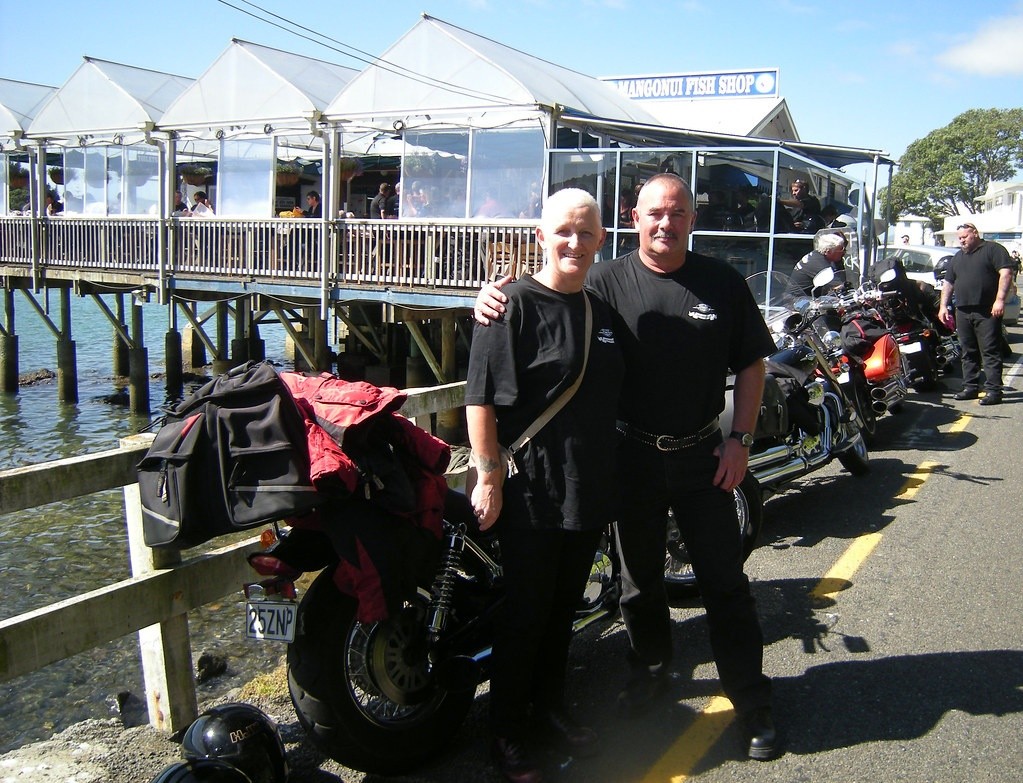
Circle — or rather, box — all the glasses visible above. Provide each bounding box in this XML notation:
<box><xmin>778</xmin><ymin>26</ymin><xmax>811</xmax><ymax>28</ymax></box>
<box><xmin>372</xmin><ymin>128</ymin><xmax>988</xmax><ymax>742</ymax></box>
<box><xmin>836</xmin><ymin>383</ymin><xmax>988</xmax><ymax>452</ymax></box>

<box><xmin>957</xmin><ymin>224</ymin><xmax>975</xmax><ymax>230</ymax></box>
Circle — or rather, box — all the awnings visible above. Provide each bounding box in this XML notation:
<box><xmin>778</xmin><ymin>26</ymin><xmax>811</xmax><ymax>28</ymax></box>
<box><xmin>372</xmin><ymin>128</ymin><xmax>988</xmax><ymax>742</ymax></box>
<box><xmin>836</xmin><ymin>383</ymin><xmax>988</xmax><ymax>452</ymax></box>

<box><xmin>552</xmin><ymin>105</ymin><xmax>901</xmax><ymax>276</ymax></box>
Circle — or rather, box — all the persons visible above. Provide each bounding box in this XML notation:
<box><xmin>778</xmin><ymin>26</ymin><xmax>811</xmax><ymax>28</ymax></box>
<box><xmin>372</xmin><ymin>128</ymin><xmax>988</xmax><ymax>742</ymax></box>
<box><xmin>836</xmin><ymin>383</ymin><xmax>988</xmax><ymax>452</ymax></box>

<box><xmin>900</xmin><ymin>235</ymin><xmax>911</xmax><ymax>245</ymax></box>
<box><xmin>404</xmin><ymin>174</ymin><xmax>541</xmax><ymax>219</ymax></box>
<box><xmin>940</xmin><ymin>223</ymin><xmax>1013</xmax><ymax>405</ymax></box>
<box><xmin>465</xmin><ymin>187</ymin><xmax>632</xmax><ymax>783</ymax></box>
<box><xmin>782</xmin><ymin>178</ymin><xmax>821</xmax><ymax>230</ymax></box>
<box><xmin>62</xmin><ymin>189</ymin><xmax>82</xmax><ymax>212</ymax></box>
<box><xmin>474</xmin><ymin>172</ymin><xmax>787</xmax><ymax>760</ymax></box>
<box><xmin>370</xmin><ymin>182</ymin><xmax>407</xmax><ymax>220</ymax></box>
<box><xmin>46</xmin><ymin>193</ymin><xmax>62</xmax><ymax>214</ymax></box>
<box><xmin>296</xmin><ymin>191</ymin><xmax>322</xmax><ymax>218</ymax></box>
<box><xmin>109</xmin><ymin>190</ymin><xmax>122</xmax><ymax>214</ymax></box>
<box><xmin>175</xmin><ymin>190</ymin><xmax>189</xmax><ymax>213</ymax></box>
<box><xmin>703</xmin><ymin>190</ymin><xmax>739</xmax><ymax>229</ymax></box>
<box><xmin>1010</xmin><ymin>250</ymin><xmax>1020</xmax><ymax>282</ymax></box>
<box><xmin>789</xmin><ymin>234</ymin><xmax>845</xmax><ymax>294</ymax></box>
<box><xmin>187</xmin><ymin>191</ymin><xmax>213</xmax><ymax>216</ymax></box>
<box><xmin>734</xmin><ymin>184</ymin><xmax>756</xmax><ymax>217</ymax></box>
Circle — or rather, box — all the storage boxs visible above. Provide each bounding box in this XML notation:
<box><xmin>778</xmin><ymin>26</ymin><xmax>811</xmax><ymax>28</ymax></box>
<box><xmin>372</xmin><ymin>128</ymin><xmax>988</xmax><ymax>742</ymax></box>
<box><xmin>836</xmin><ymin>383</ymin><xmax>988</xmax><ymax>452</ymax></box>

<box><xmin>864</xmin><ymin>332</ymin><xmax>902</xmax><ymax>381</ymax></box>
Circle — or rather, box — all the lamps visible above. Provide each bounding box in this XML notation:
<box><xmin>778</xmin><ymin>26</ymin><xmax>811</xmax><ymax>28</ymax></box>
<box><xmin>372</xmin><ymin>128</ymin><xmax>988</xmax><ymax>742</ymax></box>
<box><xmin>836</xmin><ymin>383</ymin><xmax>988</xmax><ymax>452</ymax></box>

<box><xmin>131</xmin><ymin>288</ymin><xmax>147</xmax><ymax>303</ymax></box>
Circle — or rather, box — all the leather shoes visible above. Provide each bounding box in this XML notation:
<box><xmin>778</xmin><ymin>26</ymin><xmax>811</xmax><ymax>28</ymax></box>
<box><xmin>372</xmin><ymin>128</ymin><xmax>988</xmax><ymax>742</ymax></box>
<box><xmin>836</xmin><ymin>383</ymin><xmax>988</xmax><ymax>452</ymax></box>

<box><xmin>979</xmin><ymin>392</ymin><xmax>1003</xmax><ymax>404</ymax></box>
<box><xmin>739</xmin><ymin>706</ymin><xmax>779</xmax><ymax>758</ymax></box>
<box><xmin>528</xmin><ymin>704</ymin><xmax>602</xmax><ymax>757</ymax></box>
<box><xmin>953</xmin><ymin>386</ymin><xmax>978</xmax><ymax>400</ymax></box>
<box><xmin>635</xmin><ymin>653</ymin><xmax>670</xmax><ymax>707</ymax></box>
<box><xmin>493</xmin><ymin>735</ymin><xmax>548</xmax><ymax>783</ymax></box>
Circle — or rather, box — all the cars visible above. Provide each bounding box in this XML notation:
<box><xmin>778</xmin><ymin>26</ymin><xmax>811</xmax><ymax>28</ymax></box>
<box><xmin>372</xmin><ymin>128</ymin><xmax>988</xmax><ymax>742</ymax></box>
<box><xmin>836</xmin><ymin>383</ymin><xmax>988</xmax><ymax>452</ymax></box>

<box><xmin>859</xmin><ymin>244</ymin><xmax>1021</xmax><ymax>325</ymax></box>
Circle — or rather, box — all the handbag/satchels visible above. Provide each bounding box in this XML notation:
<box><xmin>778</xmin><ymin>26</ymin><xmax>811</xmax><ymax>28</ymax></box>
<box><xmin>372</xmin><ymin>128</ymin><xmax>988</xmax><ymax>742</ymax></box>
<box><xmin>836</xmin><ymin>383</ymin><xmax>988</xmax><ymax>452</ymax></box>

<box><xmin>134</xmin><ymin>359</ymin><xmax>322</xmax><ymax>549</ymax></box>
<box><xmin>840</xmin><ymin>309</ymin><xmax>893</xmax><ymax>355</ymax></box>
<box><xmin>441</xmin><ymin>441</ymin><xmax>510</xmax><ymax>512</ymax></box>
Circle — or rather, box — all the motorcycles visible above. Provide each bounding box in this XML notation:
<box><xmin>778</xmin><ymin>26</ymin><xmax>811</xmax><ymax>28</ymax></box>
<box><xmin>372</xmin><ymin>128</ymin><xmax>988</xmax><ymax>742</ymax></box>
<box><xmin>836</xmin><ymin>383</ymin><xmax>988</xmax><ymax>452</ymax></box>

<box><xmin>241</xmin><ymin>468</ymin><xmax>763</xmax><ymax>777</ymax></box>
<box><xmin>718</xmin><ymin>267</ymin><xmax>869</xmax><ymax>487</ymax></box>
<box><xmin>820</xmin><ymin>258</ymin><xmax>1012</xmax><ymax>442</ymax></box>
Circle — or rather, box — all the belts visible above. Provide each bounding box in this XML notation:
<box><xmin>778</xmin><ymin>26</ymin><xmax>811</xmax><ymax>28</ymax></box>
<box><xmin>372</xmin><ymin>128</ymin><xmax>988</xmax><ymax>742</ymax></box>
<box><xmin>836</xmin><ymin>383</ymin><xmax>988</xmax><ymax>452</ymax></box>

<box><xmin>615</xmin><ymin>417</ymin><xmax>722</xmax><ymax>451</ymax></box>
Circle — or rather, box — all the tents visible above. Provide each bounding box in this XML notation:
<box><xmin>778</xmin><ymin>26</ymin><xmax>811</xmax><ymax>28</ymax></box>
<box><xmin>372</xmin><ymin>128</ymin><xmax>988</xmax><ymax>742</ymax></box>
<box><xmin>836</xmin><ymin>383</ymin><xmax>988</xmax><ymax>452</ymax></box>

<box><xmin>0</xmin><ymin>12</ymin><xmax>806</xmax><ymax>140</ymax></box>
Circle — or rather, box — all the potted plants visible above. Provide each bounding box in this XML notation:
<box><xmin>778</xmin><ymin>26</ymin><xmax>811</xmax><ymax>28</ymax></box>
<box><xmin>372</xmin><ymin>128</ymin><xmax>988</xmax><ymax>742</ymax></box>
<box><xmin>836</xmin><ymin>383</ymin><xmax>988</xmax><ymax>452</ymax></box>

<box><xmin>86</xmin><ymin>169</ymin><xmax>110</xmax><ymax>189</ymax></box>
<box><xmin>119</xmin><ymin>168</ymin><xmax>154</xmax><ymax>187</ymax></box>
<box><xmin>340</xmin><ymin>159</ymin><xmax>365</xmax><ymax>181</ymax></box>
<box><xmin>3</xmin><ymin>164</ymin><xmax>29</xmax><ymax>188</ymax></box>
<box><xmin>180</xmin><ymin>166</ymin><xmax>213</xmax><ymax>185</ymax></box>
<box><xmin>404</xmin><ymin>152</ymin><xmax>432</xmax><ymax>178</ymax></box>
<box><xmin>48</xmin><ymin>167</ymin><xmax>75</xmax><ymax>185</ymax></box>
<box><xmin>277</xmin><ymin>164</ymin><xmax>304</xmax><ymax>186</ymax></box>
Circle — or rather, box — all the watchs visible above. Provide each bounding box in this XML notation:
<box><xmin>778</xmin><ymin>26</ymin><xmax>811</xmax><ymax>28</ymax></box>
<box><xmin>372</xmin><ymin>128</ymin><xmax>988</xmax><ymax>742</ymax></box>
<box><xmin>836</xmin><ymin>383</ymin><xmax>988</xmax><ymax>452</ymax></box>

<box><xmin>729</xmin><ymin>431</ymin><xmax>754</xmax><ymax>447</ymax></box>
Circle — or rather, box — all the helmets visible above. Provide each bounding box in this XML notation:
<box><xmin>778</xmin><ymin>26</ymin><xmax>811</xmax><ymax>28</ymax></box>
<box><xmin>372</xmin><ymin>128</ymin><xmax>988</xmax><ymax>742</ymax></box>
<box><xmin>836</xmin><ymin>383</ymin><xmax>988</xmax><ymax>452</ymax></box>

<box><xmin>180</xmin><ymin>703</ymin><xmax>290</xmax><ymax>783</ymax></box>
<box><xmin>149</xmin><ymin>756</ymin><xmax>251</xmax><ymax>783</ymax></box>
